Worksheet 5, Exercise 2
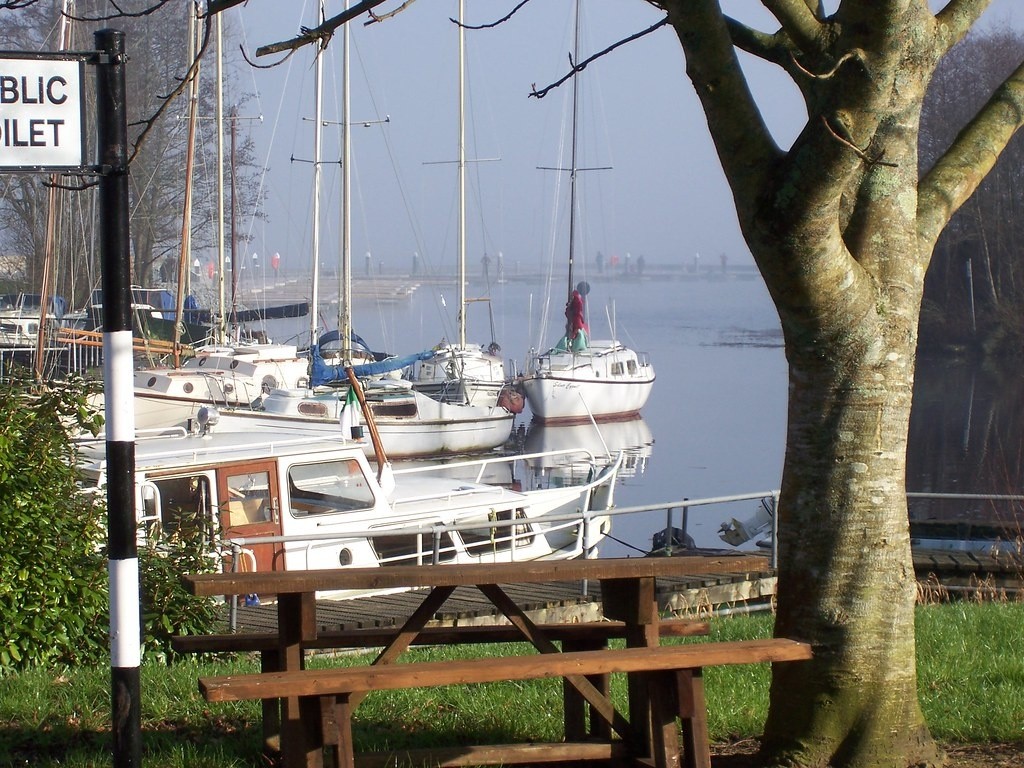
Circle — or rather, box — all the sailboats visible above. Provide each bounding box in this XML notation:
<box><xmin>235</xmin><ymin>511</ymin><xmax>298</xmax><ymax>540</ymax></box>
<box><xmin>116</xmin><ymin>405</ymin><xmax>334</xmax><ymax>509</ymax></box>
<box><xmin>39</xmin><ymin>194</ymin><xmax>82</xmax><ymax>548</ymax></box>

<box><xmin>50</xmin><ymin>1</ymin><xmax>655</xmax><ymax>606</ymax></box>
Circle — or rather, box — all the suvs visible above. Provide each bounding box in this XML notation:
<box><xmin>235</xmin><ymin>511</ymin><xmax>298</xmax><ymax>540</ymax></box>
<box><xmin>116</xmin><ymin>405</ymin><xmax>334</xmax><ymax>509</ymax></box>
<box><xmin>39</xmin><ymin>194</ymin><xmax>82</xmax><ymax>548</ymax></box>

<box><xmin>0</xmin><ymin>294</ymin><xmax>66</xmax><ymax>348</ymax></box>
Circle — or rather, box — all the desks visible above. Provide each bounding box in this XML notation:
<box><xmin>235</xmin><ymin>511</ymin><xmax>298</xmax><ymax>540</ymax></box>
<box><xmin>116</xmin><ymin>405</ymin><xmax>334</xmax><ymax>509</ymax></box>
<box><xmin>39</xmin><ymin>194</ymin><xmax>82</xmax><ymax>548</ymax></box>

<box><xmin>182</xmin><ymin>552</ymin><xmax>770</xmax><ymax>768</ymax></box>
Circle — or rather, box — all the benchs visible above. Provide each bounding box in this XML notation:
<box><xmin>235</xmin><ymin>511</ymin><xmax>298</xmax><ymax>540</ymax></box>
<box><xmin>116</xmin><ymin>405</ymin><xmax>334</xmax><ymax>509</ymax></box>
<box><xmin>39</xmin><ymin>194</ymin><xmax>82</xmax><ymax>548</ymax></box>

<box><xmin>197</xmin><ymin>637</ymin><xmax>812</xmax><ymax>768</ymax></box>
<box><xmin>170</xmin><ymin>618</ymin><xmax>711</xmax><ymax>768</ymax></box>
